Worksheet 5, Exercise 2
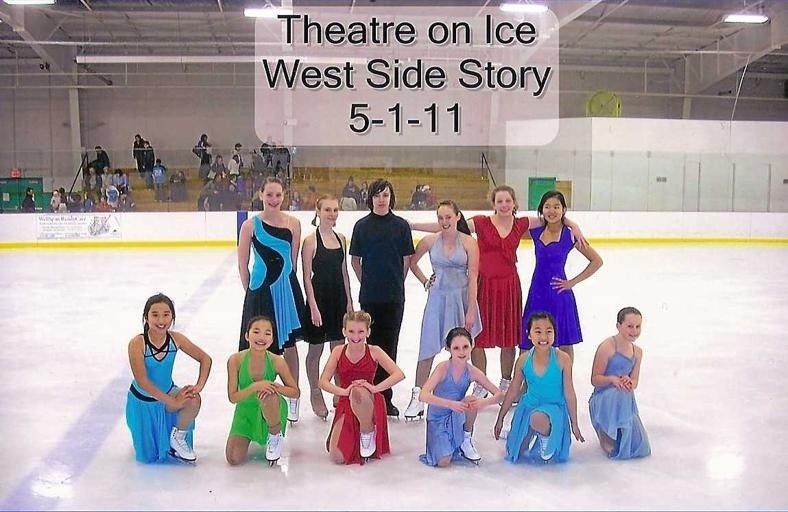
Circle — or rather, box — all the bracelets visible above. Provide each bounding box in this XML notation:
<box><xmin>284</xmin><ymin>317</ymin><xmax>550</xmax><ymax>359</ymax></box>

<box><xmin>409</xmin><ymin>221</ymin><xmax>415</xmax><ymax>231</ymax></box>
<box><xmin>423</xmin><ymin>278</ymin><xmax>428</xmax><ymax>292</ymax></box>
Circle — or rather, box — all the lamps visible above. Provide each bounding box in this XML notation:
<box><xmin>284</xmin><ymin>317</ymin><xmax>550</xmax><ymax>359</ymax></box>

<box><xmin>244</xmin><ymin>1</ymin><xmax>293</xmax><ymax>19</ymax></box>
<box><xmin>500</xmin><ymin>0</ymin><xmax>551</xmax><ymax>13</ymax></box>
<box><xmin>721</xmin><ymin>0</ymin><xmax>771</xmax><ymax>25</ymax></box>
<box><xmin>483</xmin><ymin>39</ymin><xmax>676</xmax><ymax>76</ymax></box>
<box><xmin>74</xmin><ymin>12</ymin><xmax>373</xmax><ymax>66</ymax></box>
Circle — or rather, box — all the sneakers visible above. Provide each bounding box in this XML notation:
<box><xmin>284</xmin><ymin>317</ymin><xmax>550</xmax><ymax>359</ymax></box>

<box><xmin>597</xmin><ymin>426</ymin><xmax>615</xmax><ymax>454</ymax></box>
<box><xmin>333</xmin><ymin>396</ymin><xmax>340</xmax><ymax>407</ymax></box>
<box><xmin>360</xmin><ymin>425</ymin><xmax>377</xmax><ymax>458</ymax></box>
<box><xmin>286</xmin><ymin>397</ymin><xmax>299</xmax><ymax>422</ymax></box>
<box><xmin>310</xmin><ymin>388</ymin><xmax>328</xmax><ymax>417</ymax></box>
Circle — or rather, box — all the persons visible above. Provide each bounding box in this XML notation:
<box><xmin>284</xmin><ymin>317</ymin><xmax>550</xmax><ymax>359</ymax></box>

<box><xmin>235</xmin><ymin>176</ymin><xmax>302</xmax><ymax>423</ymax></box>
<box><xmin>224</xmin><ymin>315</ymin><xmax>302</xmax><ymax>465</ymax></box>
<box><xmin>493</xmin><ymin>310</ymin><xmax>586</xmax><ymax>462</ymax></box>
<box><xmin>589</xmin><ymin>305</ymin><xmax>652</xmax><ymax>460</ymax></box>
<box><xmin>401</xmin><ymin>182</ymin><xmax>590</xmax><ymax>403</ymax></box>
<box><xmin>125</xmin><ymin>289</ymin><xmax>212</xmax><ymax>467</ymax></box>
<box><xmin>520</xmin><ymin>189</ymin><xmax>604</xmax><ymax>399</ymax></box>
<box><xmin>417</xmin><ymin>325</ymin><xmax>505</xmax><ymax>468</ymax></box>
<box><xmin>349</xmin><ymin>176</ymin><xmax>416</xmax><ymax>416</ymax></box>
<box><xmin>318</xmin><ymin>308</ymin><xmax>407</xmax><ymax>466</ymax></box>
<box><xmin>304</xmin><ymin>194</ymin><xmax>353</xmax><ymax>418</ymax></box>
<box><xmin>23</xmin><ymin>133</ymin><xmax>440</xmax><ymax>212</ymax></box>
<box><xmin>402</xmin><ymin>199</ymin><xmax>484</xmax><ymax>418</ymax></box>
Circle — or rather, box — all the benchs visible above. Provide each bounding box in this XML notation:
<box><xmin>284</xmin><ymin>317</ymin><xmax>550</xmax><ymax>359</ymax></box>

<box><xmin>78</xmin><ymin>168</ymin><xmax>497</xmax><ymax>211</ymax></box>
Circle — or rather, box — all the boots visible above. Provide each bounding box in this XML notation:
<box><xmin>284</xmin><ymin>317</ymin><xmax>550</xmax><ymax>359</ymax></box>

<box><xmin>498</xmin><ymin>378</ymin><xmax>512</xmax><ymax>406</ymax></box>
<box><xmin>459</xmin><ymin>422</ymin><xmax>481</xmax><ymax>461</ymax></box>
<box><xmin>472</xmin><ymin>380</ymin><xmax>488</xmax><ymax>398</ymax></box>
<box><xmin>404</xmin><ymin>386</ymin><xmax>424</xmax><ymax>417</ymax></box>
<box><xmin>537</xmin><ymin>433</ymin><xmax>557</xmax><ymax>461</ymax></box>
<box><xmin>169</xmin><ymin>427</ymin><xmax>196</xmax><ymax>461</ymax></box>
<box><xmin>265</xmin><ymin>431</ymin><xmax>280</xmax><ymax>461</ymax></box>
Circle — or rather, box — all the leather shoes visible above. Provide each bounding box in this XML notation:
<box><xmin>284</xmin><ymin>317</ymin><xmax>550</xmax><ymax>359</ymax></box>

<box><xmin>387</xmin><ymin>401</ymin><xmax>399</xmax><ymax>416</ymax></box>
